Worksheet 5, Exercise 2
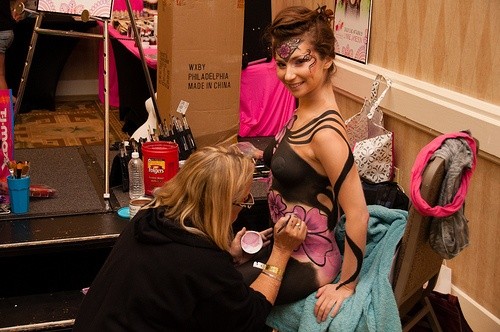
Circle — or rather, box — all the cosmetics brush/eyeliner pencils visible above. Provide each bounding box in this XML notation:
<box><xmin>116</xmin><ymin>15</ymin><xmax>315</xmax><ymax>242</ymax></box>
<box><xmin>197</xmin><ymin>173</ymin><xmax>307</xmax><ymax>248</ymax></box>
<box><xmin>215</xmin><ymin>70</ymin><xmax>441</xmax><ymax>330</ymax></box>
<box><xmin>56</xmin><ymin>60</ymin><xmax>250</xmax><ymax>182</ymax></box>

<box><xmin>4</xmin><ymin>159</ymin><xmax>32</xmax><ymax>179</ymax></box>
<box><xmin>117</xmin><ymin>113</ymin><xmax>199</xmax><ymax>194</ymax></box>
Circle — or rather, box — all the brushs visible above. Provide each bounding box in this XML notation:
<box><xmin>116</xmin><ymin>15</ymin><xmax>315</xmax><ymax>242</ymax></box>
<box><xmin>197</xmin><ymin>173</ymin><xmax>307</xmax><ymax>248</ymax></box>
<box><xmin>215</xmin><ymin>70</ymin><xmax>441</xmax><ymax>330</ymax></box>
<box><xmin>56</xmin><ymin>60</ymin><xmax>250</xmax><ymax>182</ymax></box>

<box><xmin>6</xmin><ymin>160</ymin><xmax>31</xmax><ymax>179</ymax></box>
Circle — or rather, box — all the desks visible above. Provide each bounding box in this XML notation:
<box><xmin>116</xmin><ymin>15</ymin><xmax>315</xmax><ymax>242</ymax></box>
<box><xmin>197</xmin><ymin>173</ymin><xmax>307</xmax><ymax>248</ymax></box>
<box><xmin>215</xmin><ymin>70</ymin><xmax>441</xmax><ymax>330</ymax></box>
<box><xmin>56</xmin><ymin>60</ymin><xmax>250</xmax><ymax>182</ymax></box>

<box><xmin>97</xmin><ymin>15</ymin><xmax>158</xmax><ymax>134</ymax></box>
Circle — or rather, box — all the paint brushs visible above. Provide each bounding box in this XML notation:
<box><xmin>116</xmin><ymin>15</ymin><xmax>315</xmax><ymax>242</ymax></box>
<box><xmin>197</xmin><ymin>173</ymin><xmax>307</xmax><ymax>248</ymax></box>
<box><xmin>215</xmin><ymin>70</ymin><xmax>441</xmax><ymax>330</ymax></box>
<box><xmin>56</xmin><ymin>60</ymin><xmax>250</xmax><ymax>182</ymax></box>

<box><xmin>116</xmin><ymin>113</ymin><xmax>198</xmax><ymax>192</ymax></box>
<box><xmin>276</xmin><ymin>216</ymin><xmax>292</xmax><ymax>234</ymax></box>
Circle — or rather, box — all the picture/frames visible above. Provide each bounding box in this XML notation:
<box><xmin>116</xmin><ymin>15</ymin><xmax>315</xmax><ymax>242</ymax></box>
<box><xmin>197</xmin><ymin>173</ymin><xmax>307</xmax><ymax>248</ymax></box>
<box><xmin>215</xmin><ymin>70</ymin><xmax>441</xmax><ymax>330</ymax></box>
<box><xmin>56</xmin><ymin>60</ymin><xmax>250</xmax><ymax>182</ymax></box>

<box><xmin>333</xmin><ymin>0</ymin><xmax>372</xmax><ymax>65</ymax></box>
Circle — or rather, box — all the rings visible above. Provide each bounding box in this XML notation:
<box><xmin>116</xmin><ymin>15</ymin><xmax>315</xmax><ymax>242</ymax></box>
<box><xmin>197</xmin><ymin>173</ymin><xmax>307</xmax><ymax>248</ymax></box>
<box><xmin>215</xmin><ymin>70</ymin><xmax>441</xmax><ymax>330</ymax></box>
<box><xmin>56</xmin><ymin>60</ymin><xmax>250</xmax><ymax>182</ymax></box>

<box><xmin>295</xmin><ymin>222</ymin><xmax>301</xmax><ymax>230</ymax></box>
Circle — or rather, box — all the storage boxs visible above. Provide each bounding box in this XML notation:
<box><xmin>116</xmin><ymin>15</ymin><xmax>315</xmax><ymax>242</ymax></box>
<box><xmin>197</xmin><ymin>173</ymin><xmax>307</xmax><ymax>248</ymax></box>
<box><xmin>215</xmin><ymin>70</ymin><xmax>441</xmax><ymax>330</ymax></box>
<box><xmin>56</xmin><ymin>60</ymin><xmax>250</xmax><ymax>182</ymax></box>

<box><xmin>157</xmin><ymin>0</ymin><xmax>245</xmax><ymax>153</ymax></box>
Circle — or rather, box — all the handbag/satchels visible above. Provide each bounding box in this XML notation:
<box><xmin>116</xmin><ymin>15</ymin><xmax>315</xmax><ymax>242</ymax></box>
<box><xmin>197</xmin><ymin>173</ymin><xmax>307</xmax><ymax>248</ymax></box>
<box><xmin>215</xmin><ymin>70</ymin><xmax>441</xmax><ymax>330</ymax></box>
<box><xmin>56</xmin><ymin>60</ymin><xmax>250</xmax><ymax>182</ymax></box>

<box><xmin>346</xmin><ymin>74</ymin><xmax>394</xmax><ymax>184</ymax></box>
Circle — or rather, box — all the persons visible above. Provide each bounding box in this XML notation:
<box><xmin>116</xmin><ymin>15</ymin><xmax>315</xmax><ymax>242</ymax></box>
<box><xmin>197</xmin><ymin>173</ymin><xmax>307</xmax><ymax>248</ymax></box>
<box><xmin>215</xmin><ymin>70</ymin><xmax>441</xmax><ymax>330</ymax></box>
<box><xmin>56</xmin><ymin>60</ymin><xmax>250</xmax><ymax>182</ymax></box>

<box><xmin>229</xmin><ymin>4</ymin><xmax>370</xmax><ymax>324</ymax></box>
<box><xmin>0</xmin><ymin>0</ymin><xmax>18</xmax><ymax>112</ymax></box>
<box><xmin>73</xmin><ymin>143</ymin><xmax>308</xmax><ymax>332</ymax></box>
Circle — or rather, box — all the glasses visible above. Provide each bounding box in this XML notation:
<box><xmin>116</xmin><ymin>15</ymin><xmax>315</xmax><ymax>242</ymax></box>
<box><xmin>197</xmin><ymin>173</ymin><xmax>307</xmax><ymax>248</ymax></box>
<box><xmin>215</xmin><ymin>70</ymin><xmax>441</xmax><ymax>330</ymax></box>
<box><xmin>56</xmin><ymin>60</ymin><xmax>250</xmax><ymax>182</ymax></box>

<box><xmin>232</xmin><ymin>192</ymin><xmax>255</xmax><ymax>209</ymax></box>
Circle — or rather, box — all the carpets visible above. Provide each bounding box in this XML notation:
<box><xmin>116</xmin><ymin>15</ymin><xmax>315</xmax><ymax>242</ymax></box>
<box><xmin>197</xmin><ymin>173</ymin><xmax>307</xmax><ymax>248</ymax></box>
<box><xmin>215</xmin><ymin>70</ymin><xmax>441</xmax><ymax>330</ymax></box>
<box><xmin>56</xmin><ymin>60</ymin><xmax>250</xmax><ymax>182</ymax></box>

<box><xmin>0</xmin><ymin>144</ymin><xmax>131</xmax><ymax>218</ymax></box>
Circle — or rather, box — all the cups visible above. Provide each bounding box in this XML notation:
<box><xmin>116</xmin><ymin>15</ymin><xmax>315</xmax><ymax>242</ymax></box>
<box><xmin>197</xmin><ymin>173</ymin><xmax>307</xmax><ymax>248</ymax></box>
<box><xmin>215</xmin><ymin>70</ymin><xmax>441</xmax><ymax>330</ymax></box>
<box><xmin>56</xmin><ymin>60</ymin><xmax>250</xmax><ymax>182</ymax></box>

<box><xmin>7</xmin><ymin>176</ymin><xmax>31</xmax><ymax>215</ymax></box>
<box><xmin>129</xmin><ymin>198</ymin><xmax>153</xmax><ymax>219</ymax></box>
<box><xmin>240</xmin><ymin>230</ymin><xmax>263</xmax><ymax>254</ymax></box>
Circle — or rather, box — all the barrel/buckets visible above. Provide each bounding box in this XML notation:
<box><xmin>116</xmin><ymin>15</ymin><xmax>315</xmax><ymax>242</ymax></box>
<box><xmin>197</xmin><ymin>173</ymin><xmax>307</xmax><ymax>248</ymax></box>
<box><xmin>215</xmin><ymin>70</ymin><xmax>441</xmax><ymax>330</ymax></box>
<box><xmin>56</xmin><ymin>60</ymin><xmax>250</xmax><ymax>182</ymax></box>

<box><xmin>141</xmin><ymin>141</ymin><xmax>179</xmax><ymax>195</ymax></box>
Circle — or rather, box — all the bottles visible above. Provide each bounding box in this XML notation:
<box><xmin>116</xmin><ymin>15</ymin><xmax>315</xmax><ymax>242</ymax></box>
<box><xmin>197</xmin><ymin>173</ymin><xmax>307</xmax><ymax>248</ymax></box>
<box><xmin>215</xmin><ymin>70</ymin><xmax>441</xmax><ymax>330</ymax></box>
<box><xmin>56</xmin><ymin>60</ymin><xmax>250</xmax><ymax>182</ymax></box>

<box><xmin>128</xmin><ymin>152</ymin><xmax>145</xmax><ymax>200</ymax></box>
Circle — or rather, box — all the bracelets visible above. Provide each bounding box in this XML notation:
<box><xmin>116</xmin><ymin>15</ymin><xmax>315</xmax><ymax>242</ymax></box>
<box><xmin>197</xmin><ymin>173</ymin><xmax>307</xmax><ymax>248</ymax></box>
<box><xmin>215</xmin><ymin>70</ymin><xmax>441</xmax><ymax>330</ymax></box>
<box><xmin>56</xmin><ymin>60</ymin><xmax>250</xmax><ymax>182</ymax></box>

<box><xmin>261</xmin><ymin>270</ymin><xmax>283</xmax><ymax>284</ymax></box>
<box><xmin>264</xmin><ymin>263</ymin><xmax>286</xmax><ymax>278</ymax></box>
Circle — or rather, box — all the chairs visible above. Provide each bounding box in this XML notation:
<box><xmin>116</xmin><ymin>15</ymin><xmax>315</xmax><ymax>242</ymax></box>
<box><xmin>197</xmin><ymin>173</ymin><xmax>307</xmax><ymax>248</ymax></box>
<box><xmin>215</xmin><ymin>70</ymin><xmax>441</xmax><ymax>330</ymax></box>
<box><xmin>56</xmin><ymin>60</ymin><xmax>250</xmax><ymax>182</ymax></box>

<box><xmin>391</xmin><ymin>129</ymin><xmax>480</xmax><ymax>332</ymax></box>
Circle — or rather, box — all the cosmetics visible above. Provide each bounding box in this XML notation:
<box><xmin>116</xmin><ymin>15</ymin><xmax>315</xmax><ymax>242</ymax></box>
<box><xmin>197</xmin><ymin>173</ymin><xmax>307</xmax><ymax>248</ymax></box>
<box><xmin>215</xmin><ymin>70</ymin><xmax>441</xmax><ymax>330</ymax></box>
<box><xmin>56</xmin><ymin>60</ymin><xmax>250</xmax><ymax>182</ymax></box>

<box><xmin>111</xmin><ymin>8</ymin><xmax>157</xmax><ymax>50</ymax></box>
